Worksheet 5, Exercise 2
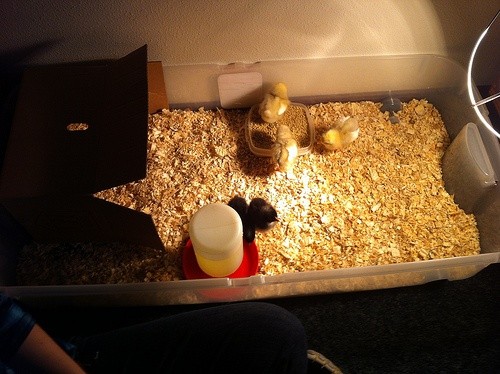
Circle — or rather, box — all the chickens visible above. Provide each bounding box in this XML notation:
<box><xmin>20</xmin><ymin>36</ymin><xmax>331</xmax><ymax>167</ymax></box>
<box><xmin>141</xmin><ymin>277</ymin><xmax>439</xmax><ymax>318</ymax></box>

<box><xmin>258</xmin><ymin>80</ymin><xmax>291</xmax><ymax>124</ymax></box>
<box><xmin>317</xmin><ymin>115</ymin><xmax>360</xmax><ymax>151</ymax></box>
<box><xmin>271</xmin><ymin>125</ymin><xmax>298</xmax><ymax>174</ymax></box>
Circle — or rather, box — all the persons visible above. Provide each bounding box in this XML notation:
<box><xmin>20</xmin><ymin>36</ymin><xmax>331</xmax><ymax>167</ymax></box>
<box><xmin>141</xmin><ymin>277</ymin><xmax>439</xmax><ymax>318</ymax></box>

<box><xmin>1</xmin><ymin>291</ymin><xmax>310</xmax><ymax>374</ymax></box>
